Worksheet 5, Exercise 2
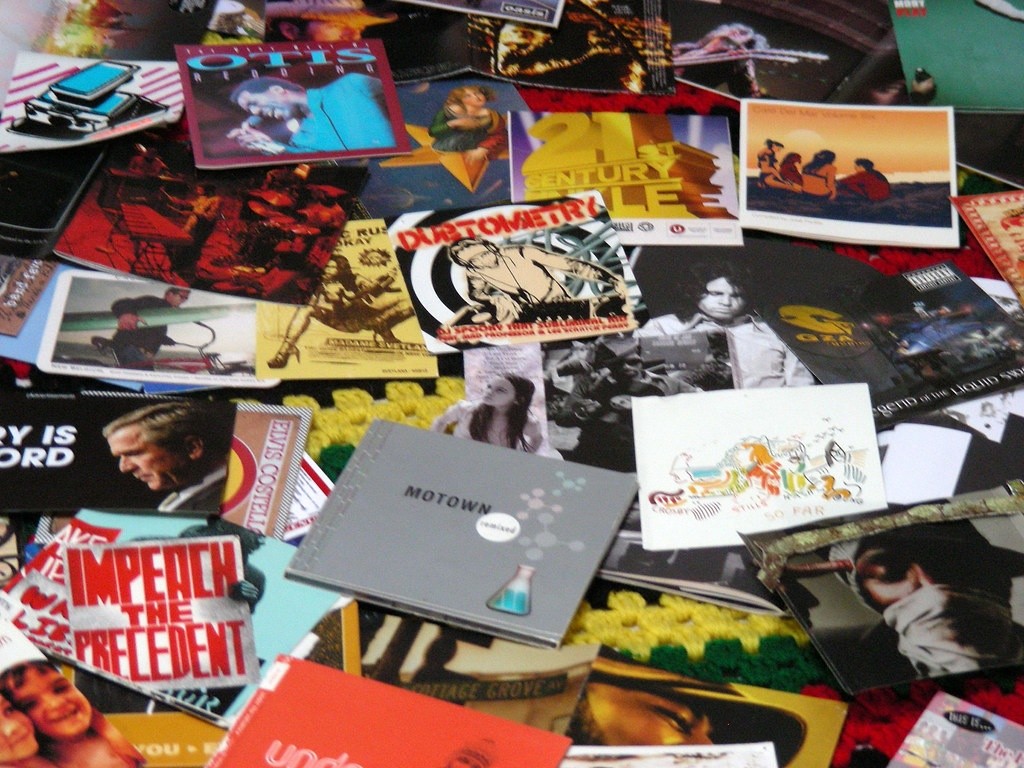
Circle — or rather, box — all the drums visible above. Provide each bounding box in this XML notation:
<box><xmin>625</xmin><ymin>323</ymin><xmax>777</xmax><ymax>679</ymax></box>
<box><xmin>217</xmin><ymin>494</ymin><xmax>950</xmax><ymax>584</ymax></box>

<box><xmin>270</xmin><ymin>215</ymin><xmax>291</xmax><ymax>240</ymax></box>
<box><xmin>288</xmin><ymin>224</ymin><xmax>322</xmax><ymax>249</ymax></box>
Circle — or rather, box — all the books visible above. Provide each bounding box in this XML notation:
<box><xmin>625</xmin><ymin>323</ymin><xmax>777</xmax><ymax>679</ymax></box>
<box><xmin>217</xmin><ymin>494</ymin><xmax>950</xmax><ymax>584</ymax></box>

<box><xmin>0</xmin><ymin>0</ymin><xmax>1024</xmax><ymax>768</ymax></box>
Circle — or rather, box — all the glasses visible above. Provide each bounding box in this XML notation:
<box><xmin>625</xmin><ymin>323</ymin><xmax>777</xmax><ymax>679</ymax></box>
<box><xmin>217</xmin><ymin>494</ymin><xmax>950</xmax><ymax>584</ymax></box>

<box><xmin>177</xmin><ymin>292</ymin><xmax>188</xmax><ymax>302</ymax></box>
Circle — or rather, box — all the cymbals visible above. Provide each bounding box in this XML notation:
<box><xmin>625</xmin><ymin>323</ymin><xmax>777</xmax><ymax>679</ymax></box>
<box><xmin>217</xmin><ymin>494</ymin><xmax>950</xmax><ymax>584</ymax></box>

<box><xmin>262</xmin><ymin>190</ymin><xmax>294</xmax><ymax>207</ymax></box>
<box><xmin>250</xmin><ymin>201</ymin><xmax>282</xmax><ymax>220</ymax></box>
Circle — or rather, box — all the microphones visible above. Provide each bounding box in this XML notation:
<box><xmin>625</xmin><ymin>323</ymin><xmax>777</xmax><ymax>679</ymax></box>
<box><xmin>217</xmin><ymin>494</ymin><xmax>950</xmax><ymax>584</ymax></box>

<box><xmin>234</xmin><ymin>115</ymin><xmax>264</xmax><ymax>142</ymax></box>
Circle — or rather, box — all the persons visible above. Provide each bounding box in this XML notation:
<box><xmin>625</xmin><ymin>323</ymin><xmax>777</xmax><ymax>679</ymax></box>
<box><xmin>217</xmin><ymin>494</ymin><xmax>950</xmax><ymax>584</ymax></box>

<box><xmin>639</xmin><ymin>262</ymin><xmax>817</xmax><ymax>393</ymax></box>
<box><xmin>0</xmin><ymin>659</ymin><xmax>137</xmax><ymax>768</ymax></box>
<box><xmin>167</xmin><ymin>181</ymin><xmax>220</xmax><ymax>265</ymax></box>
<box><xmin>0</xmin><ymin>693</ymin><xmax>58</xmax><ymax>768</ymax></box>
<box><xmin>102</xmin><ymin>399</ymin><xmax>227</xmax><ymax>513</ymax></box>
<box><xmin>107</xmin><ymin>287</ymin><xmax>192</xmax><ymax>371</ymax></box>
<box><xmin>223</xmin><ymin>71</ymin><xmax>398</xmax><ymax>156</ymax></box>
<box><xmin>570</xmin><ymin>659</ymin><xmax>808</xmax><ymax>758</ymax></box>
<box><xmin>123</xmin><ymin>146</ymin><xmax>173</xmax><ymax>181</ymax></box>
<box><xmin>840</xmin><ymin>515</ymin><xmax>1024</xmax><ymax>675</ymax></box>
<box><xmin>432</xmin><ymin>371</ymin><xmax>542</xmax><ymax>456</ymax></box>
